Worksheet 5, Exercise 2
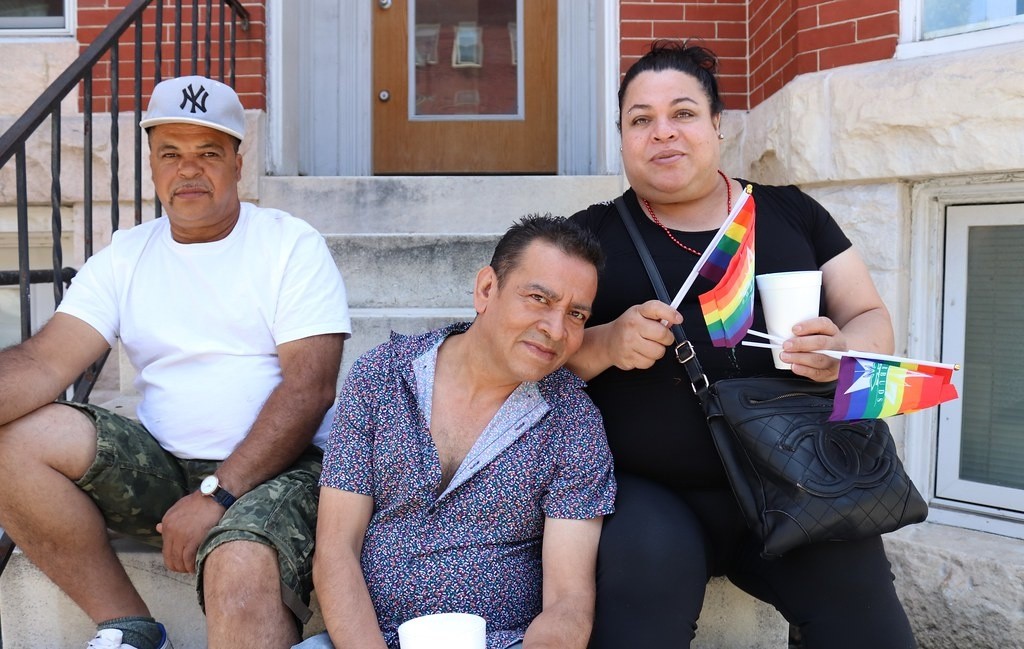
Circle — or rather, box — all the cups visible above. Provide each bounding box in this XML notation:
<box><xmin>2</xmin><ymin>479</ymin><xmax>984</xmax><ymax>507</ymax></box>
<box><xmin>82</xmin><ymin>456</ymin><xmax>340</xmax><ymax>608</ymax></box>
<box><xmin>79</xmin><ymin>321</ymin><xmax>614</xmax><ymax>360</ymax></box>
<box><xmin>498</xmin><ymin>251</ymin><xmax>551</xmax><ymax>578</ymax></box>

<box><xmin>755</xmin><ymin>270</ymin><xmax>823</xmax><ymax>369</ymax></box>
<box><xmin>396</xmin><ymin>613</ymin><xmax>488</xmax><ymax>649</ymax></box>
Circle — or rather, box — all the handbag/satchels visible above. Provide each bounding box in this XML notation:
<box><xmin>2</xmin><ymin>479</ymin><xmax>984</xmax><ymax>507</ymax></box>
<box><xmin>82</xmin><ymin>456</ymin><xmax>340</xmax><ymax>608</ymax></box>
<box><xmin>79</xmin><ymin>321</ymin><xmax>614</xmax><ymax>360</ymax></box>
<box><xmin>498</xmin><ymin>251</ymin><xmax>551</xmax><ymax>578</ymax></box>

<box><xmin>698</xmin><ymin>375</ymin><xmax>929</xmax><ymax>559</ymax></box>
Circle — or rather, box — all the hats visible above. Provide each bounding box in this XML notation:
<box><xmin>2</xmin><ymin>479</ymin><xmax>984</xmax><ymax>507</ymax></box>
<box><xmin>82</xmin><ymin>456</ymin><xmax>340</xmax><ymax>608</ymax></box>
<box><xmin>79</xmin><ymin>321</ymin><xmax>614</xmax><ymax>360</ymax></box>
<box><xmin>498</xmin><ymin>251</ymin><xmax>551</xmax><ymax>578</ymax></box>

<box><xmin>138</xmin><ymin>74</ymin><xmax>247</xmax><ymax>141</ymax></box>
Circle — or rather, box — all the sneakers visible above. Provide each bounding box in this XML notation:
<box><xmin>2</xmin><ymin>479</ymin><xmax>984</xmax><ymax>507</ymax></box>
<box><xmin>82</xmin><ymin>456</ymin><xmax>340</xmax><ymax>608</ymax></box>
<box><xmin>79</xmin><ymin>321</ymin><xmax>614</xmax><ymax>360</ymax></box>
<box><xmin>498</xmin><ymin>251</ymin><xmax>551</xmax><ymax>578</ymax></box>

<box><xmin>86</xmin><ymin>619</ymin><xmax>176</xmax><ymax>649</ymax></box>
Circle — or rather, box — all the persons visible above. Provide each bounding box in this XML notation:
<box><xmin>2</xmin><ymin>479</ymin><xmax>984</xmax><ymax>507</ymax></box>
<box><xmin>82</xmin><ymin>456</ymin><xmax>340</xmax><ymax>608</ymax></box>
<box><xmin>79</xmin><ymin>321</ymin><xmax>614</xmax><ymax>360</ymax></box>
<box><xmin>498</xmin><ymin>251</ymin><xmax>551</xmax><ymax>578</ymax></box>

<box><xmin>0</xmin><ymin>75</ymin><xmax>353</xmax><ymax>649</ymax></box>
<box><xmin>568</xmin><ymin>35</ymin><xmax>921</xmax><ymax>649</ymax></box>
<box><xmin>292</xmin><ymin>210</ymin><xmax>619</xmax><ymax>649</ymax></box>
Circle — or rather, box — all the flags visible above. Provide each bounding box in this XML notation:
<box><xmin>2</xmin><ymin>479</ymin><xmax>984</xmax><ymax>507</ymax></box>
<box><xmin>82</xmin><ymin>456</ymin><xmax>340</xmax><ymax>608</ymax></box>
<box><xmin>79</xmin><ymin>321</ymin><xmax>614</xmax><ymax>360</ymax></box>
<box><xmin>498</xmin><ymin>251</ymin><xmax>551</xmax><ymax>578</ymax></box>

<box><xmin>698</xmin><ymin>195</ymin><xmax>756</xmax><ymax>348</ymax></box>
<box><xmin>826</xmin><ymin>355</ymin><xmax>960</xmax><ymax>421</ymax></box>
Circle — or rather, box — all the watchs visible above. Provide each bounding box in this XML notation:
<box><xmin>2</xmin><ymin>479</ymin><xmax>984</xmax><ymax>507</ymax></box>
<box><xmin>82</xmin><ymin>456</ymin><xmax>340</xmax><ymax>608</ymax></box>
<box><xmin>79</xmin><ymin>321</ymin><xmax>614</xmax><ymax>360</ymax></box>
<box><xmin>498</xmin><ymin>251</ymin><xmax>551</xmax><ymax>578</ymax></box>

<box><xmin>200</xmin><ymin>474</ymin><xmax>237</xmax><ymax>510</ymax></box>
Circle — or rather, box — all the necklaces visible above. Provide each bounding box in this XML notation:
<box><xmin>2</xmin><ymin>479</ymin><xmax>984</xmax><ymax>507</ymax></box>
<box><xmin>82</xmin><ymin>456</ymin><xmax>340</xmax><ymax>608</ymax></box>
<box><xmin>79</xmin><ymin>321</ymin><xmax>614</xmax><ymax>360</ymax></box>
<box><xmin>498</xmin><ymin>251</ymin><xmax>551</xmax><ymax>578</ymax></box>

<box><xmin>641</xmin><ymin>169</ymin><xmax>732</xmax><ymax>256</ymax></box>
